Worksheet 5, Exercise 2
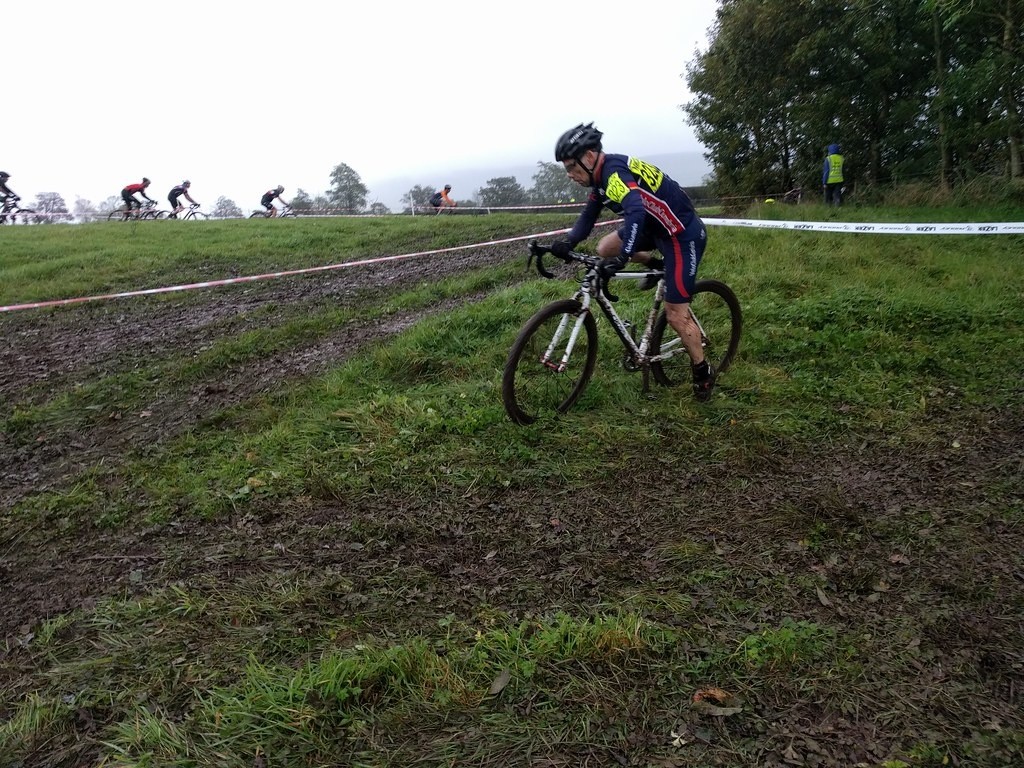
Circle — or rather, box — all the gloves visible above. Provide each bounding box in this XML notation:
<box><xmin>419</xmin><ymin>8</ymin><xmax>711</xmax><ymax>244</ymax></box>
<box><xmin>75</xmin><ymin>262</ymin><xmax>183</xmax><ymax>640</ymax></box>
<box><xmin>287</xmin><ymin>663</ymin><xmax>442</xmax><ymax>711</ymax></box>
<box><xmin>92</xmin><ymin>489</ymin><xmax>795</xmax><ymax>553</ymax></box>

<box><xmin>597</xmin><ymin>254</ymin><xmax>627</xmax><ymax>277</ymax></box>
<box><xmin>552</xmin><ymin>237</ymin><xmax>575</xmax><ymax>265</ymax></box>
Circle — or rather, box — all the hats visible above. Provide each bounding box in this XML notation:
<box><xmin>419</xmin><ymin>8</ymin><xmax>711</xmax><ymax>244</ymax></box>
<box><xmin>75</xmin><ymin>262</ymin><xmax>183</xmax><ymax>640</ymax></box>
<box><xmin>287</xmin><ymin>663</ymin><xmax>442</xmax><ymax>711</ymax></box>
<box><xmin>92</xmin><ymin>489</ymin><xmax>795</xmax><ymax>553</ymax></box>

<box><xmin>827</xmin><ymin>143</ymin><xmax>838</xmax><ymax>154</ymax></box>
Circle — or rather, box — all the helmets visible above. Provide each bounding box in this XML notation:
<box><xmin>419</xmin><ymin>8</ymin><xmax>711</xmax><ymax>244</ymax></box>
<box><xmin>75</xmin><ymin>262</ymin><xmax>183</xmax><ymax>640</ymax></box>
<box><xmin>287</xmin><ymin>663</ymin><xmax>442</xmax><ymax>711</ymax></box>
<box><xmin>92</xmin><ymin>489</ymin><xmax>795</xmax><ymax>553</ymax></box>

<box><xmin>183</xmin><ymin>179</ymin><xmax>191</xmax><ymax>184</ymax></box>
<box><xmin>0</xmin><ymin>172</ymin><xmax>10</xmax><ymax>178</ymax></box>
<box><xmin>445</xmin><ymin>185</ymin><xmax>451</xmax><ymax>189</ymax></box>
<box><xmin>278</xmin><ymin>185</ymin><xmax>284</xmax><ymax>191</ymax></box>
<box><xmin>555</xmin><ymin>121</ymin><xmax>602</xmax><ymax>160</ymax></box>
<box><xmin>143</xmin><ymin>178</ymin><xmax>150</xmax><ymax>184</ymax></box>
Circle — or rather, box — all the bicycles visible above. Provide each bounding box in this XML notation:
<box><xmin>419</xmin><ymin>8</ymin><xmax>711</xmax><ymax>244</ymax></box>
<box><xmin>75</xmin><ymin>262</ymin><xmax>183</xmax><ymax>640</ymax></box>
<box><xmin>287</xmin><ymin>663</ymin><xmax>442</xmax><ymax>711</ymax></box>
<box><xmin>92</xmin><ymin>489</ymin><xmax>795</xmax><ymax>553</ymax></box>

<box><xmin>0</xmin><ymin>194</ymin><xmax>40</xmax><ymax>226</ymax></box>
<box><xmin>154</xmin><ymin>204</ymin><xmax>210</xmax><ymax>220</ymax></box>
<box><xmin>108</xmin><ymin>197</ymin><xmax>157</xmax><ymax>221</ymax></box>
<box><xmin>428</xmin><ymin>203</ymin><xmax>461</xmax><ymax>215</ymax></box>
<box><xmin>248</xmin><ymin>203</ymin><xmax>298</xmax><ymax>218</ymax></box>
<box><xmin>500</xmin><ymin>240</ymin><xmax>744</xmax><ymax>428</ymax></box>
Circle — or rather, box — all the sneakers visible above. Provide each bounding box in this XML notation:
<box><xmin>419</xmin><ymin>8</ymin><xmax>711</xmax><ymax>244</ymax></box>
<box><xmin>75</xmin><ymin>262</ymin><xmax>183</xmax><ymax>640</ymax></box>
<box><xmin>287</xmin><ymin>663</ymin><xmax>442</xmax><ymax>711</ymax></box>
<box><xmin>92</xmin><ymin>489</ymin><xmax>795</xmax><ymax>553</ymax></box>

<box><xmin>641</xmin><ymin>263</ymin><xmax>665</xmax><ymax>291</ymax></box>
<box><xmin>694</xmin><ymin>367</ymin><xmax>716</xmax><ymax>403</ymax></box>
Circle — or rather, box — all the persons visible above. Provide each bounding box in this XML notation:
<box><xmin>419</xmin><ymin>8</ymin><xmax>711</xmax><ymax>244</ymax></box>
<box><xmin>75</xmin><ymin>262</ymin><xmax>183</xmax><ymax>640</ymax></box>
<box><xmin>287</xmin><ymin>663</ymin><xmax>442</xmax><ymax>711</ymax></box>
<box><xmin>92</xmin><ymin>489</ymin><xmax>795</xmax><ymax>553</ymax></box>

<box><xmin>260</xmin><ymin>185</ymin><xmax>289</xmax><ymax>219</ymax></box>
<box><xmin>430</xmin><ymin>184</ymin><xmax>457</xmax><ymax>216</ymax></box>
<box><xmin>168</xmin><ymin>181</ymin><xmax>197</xmax><ymax>219</ymax></box>
<box><xmin>0</xmin><ymin>168</ymin><xmax>22</xmax><ymax>228</ymax></box>
<box><xmin>550</xmin><ymin>121</ymin><xmax>718</xmax><ymax>405</ymax></box>
<box><xmin>822</xmin><ymin>143</ymin><xmax>846</xmax><ymax>211</ymax></box>
<box><xmin>121</xmin><ymin>178</ymin><xmax>153</xmax><ymax>219</ymax></box>
